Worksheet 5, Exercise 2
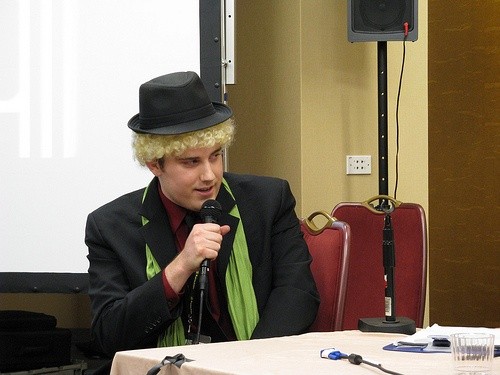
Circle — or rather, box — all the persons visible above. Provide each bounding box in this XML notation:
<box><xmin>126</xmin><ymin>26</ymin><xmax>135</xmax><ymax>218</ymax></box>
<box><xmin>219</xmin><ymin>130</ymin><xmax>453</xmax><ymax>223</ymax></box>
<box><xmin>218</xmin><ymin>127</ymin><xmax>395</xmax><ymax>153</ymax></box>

<box><xmin>83</xmin><ymin>71</ymin><xmax>322</xmax><ymax>375</ymax></box>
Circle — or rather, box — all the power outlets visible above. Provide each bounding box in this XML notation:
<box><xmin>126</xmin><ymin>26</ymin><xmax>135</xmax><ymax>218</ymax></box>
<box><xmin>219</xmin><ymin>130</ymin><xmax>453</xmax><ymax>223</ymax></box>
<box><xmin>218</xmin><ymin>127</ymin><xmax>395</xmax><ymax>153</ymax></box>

<box><xmin>346</xmin><ymin>155</ymin><xmax>372</xmax><ymax>175</ymax></box>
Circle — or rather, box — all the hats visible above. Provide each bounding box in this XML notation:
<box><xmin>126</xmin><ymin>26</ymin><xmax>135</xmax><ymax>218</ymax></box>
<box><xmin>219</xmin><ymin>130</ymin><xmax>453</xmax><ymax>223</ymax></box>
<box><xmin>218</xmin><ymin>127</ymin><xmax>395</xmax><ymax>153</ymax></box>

<box><xmin>127</xmin><ymin>71</ymin><xmax>232</xmax><ymax>135</ymax></box>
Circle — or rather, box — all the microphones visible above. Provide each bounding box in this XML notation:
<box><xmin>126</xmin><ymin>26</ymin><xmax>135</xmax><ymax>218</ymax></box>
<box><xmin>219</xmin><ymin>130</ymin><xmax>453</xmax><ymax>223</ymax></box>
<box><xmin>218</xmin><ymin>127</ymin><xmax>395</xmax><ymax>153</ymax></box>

<box><xmin>199</xmin><ymin>200</ymin><xmax>223</xmax><ymax>290</ymax></box>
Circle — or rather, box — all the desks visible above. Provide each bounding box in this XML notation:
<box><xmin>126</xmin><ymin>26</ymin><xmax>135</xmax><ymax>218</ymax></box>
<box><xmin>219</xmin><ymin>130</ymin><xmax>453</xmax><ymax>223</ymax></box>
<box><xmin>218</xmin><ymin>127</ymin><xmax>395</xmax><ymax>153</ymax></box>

<box><xmin>108</xmin><ymin>329</ymin><xmax>500</xmax><ymax>375</ymax></box>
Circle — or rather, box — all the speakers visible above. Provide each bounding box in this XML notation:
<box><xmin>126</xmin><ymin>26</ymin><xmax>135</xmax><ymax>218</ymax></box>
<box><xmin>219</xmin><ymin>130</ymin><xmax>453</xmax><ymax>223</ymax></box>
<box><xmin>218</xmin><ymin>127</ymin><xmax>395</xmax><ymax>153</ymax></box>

<box><xmin>346</xmin><ymin>0</ymin><xmax>418</xmax><ymax>43</ymax></box>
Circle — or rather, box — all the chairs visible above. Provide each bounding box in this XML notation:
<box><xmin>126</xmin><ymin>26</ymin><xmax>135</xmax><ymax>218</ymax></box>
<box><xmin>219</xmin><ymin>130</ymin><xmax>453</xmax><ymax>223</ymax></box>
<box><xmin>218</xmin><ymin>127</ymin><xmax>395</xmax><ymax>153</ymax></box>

<box><xmin>297</xmin><ymin>209</ymin><xmax>351</xmax><ymax>334</ymax></box>
<box><xmin>332</xmin><ymin>194</ymin><xmax>427</xmax><ymax>330</ymax></box>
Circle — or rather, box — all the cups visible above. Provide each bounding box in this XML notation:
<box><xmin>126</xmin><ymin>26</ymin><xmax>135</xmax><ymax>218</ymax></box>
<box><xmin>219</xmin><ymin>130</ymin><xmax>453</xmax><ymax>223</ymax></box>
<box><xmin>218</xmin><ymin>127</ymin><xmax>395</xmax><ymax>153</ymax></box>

<box><xmin>450</xmin><ymin>333</ymin><xmax>495</xmax><ymax>375</ymax></box>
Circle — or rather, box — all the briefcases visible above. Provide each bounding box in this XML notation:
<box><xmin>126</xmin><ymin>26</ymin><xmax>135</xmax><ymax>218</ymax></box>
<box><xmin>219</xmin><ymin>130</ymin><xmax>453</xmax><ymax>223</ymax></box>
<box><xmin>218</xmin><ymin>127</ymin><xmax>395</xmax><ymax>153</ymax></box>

<box><xmin>0</xmin><ymin>328</ymin><xmax>71</xmax><ymax>373</ymax></box>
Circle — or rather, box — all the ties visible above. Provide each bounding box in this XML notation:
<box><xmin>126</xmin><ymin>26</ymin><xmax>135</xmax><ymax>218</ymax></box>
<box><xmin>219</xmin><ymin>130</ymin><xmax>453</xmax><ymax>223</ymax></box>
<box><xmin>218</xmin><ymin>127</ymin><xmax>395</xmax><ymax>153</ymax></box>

<box><xmin>184</xmin><ymin>211</ymin><xmax>211</xmax><ymax>310</ymax></box>
